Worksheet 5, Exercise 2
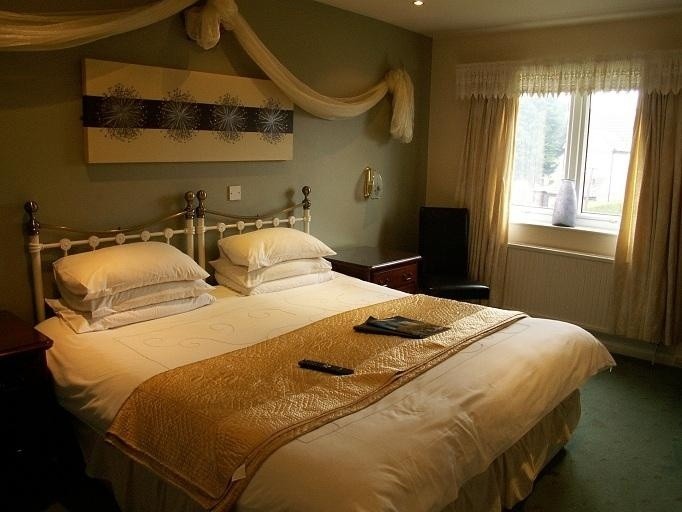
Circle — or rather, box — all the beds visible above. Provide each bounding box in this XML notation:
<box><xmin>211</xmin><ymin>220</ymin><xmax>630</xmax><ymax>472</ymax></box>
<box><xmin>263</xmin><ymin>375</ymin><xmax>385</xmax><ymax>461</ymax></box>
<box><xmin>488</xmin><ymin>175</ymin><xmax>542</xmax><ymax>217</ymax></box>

<box><xmin>25</xmin><ymin>184</ymin><xmax>618</xmax><ymax>511</ymax></box>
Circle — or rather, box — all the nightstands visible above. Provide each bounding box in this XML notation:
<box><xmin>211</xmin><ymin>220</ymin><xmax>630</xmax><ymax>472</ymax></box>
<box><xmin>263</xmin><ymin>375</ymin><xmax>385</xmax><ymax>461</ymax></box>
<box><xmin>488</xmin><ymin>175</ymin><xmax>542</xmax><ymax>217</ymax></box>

<box><xmin>0</xmin><ymin>311</ymin><xmax>53</xmax><ymax>512</ymax></box>
<box><xmin>323</xmin><ymin>246</ymin><xmax>421</xmax><ymax>294</ymax></box>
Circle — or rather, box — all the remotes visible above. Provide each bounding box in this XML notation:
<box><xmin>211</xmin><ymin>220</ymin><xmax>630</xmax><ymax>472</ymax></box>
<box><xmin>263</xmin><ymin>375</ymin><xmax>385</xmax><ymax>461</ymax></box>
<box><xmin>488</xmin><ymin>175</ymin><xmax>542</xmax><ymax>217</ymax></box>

<box><xmin>298</xmin><ymin>359</ymin><xmax>354</xmax><ymax>375</ymax></box>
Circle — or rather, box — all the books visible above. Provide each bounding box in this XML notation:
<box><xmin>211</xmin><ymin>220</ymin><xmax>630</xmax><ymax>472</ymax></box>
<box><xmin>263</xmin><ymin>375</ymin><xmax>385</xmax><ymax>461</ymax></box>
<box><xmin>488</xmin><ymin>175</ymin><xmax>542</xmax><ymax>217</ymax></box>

<box><xmin>354</xmin><ymin>315</ymin><xmax>450</xmax><ymax>340</ymax></box>
<box><xmin>368</xmin><ymin>315</ymin><xmax>446</xmax><ymax>336</ymax></box>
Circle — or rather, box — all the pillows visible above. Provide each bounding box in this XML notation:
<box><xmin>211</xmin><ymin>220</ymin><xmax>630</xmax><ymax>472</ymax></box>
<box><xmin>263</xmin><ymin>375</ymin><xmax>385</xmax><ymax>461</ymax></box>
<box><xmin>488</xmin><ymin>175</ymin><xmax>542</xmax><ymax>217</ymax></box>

<box><xmin>44</xmin><ymin>226</ymin><xmax>338</xmax><ymax>334</ymax></box>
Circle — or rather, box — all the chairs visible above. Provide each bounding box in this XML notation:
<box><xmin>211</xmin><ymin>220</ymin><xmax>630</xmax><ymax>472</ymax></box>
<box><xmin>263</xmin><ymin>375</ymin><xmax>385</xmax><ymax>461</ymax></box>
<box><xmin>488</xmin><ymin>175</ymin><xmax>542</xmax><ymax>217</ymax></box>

<box><xmin>418</xmin><ymin>207</ymin><xmax>490</xmax><ymax>306</ymax></box>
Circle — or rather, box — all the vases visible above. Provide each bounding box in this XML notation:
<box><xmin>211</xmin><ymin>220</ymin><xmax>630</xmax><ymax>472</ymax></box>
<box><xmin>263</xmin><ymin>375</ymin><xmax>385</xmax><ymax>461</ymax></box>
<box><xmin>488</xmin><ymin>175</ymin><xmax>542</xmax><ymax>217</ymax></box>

<box><xmin>553</xmin><ymin>179</ymin><xmax>577</xmax><ymax>227</ymax></box>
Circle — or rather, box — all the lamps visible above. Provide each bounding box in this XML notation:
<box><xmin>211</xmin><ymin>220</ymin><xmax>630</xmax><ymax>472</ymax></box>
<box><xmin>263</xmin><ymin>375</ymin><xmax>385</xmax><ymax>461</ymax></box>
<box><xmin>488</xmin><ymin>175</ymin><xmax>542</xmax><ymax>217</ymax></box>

<box><xmin>364</xmin><ymin>168</ymin><xmax>384</xmax><ymax>200</ymax></box>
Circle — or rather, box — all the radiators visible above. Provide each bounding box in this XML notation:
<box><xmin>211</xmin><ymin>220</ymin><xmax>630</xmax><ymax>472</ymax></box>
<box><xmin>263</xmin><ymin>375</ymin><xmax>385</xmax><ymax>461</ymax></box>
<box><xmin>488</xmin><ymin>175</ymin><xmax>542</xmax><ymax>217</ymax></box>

<box><xmin>502</xmin><ymin>242</ymin><xmax>617</xmax><ymax>335</ymax></box>
